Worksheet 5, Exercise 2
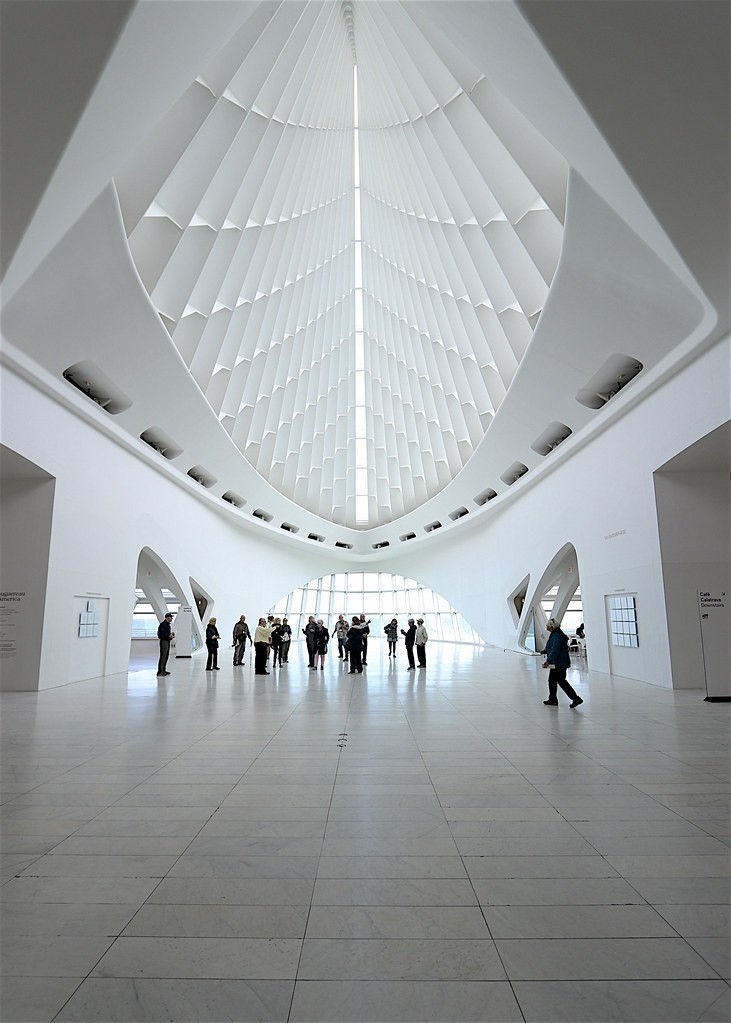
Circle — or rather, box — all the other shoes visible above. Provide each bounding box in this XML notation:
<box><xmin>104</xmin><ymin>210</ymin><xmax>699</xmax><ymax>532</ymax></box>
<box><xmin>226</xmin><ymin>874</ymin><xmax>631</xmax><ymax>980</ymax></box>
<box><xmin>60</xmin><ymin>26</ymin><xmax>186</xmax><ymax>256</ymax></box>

<box><xmin>570</xmin><ymin>696</ymin><xmax>583</xmax><ymax>708</ymax></box>
<box><xmin>363</xmin><ymin>661</ymin><xmax>367</xmax><ymax>665</ymax></box>
<box><xmin>234</xmin><ymin>662</ymin><xmax>238</xmax><ymax>666</ymax></box>
<box><xmin>343</xmin><ymin>658</ymin><xmax>348</xmax><ymax>661</ymax></box>
<box><xmin>255</xmin><ymin>670</ymin><xmax>270</xmax><ymax>675</ymax></box>
<box><xmin>213</xmin><ymin>666</ymin><xmax>220</xmax><ymax>670</ymax></box>
<box><xmin>389</xmin><ymin>651</ymin><xmax>392</xmax><ymax>656</ymax></box>
<box><xmin>157</xmin><ymin>671</ymin><xmax>171</xmax><ymax>676</ymax></box>
<box><xmin>393</xmin><ymin>655</ymin><xmax>396</xmax><ymax>657</ymax></box>
<box><xmin>206</xmin><ymin>668</ymin><xmax>213</xmax><ymax>670</ymax></box>
<box><xmin>543</xmin><ymin>699</ymin><xmax>558</xmax><ymax>706</ymax></box>
<box><xmin>339</xmin><ymin>655</ymin><xmax>343</xmax><ymax>658</ymax></box>
<box><xmin>407</xmin><ymin>667</ymin><xmax>415</xmax><ymax>670</ymax></box>
<box><xmin>348</xmin><ymin>670</ymin><xmax>355</xmax><ymax>674</ymax></box>
<box><xmin>358</xmin><ymin>668</ymin><xmax>363</xmax><ymax>673</ymax></box>
<box><xmin>417</xmin><ymin>664</ymin><xmax>426</xmax><ymax>668</ymax></box>
<box><xmin>238</xmin><ymin>662</ymin><xmax>245</xmax><ymax>665</ymax></box>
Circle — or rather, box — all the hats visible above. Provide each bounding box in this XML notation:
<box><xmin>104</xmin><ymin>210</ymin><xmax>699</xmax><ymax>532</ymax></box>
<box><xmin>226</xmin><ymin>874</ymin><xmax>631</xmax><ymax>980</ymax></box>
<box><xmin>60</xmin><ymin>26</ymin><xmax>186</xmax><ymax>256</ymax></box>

<box><xmin>416</xmin><ymin>619</ymin><xmax>424</xmax><ymax>623</ymax></box>
<box><xmin>165</xmin><ymin>613</ymin><xmax>172</xmax><ymax>617</ymax></box>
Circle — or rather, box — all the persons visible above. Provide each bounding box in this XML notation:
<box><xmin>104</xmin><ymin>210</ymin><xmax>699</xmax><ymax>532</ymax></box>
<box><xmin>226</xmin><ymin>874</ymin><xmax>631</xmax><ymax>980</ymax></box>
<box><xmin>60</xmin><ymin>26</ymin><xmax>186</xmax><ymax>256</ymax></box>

<box><xmin>539</xmin><ymin>618</ymin><xmax>584</xmax><ymax>708</ymax></box>
<box><xmin>302</xmin><ymin>616</ymin><xmax>318</xmax><ymax>667</ymax></box>
<box><xmin>415</xmin><ymin>618</ymin><xmax>428</xmax><ymax>668</ymax></box>
<box><xmin>384</xmin><ymin>618</ymin><xmax>398</xmax><ymax>658</ymax></box>
<box><xmin>346</xmin><ymin>616</ymin><xmax>363</xmax><ymax>674</ymax></box>
<box><xmin>271</xmin><ymin>618</ymin><xmax>284</xmax><ymax>668</ymax></box>
<box><xmin>205</xmin><ymin>618</ymin><xmax>220</xmax><ymax>671</ymax></box>
<box><xmin>233</xmin><ymin>615</ymin><xmax>253</xmax><ymax>666</ymax></box>
<box><xmin>263</xmin><ymin>616</ymin><xmax>274</xmax><ymax>660</ymax></box>
<box><xmin>569</xmin><ymin>623</ymin><xmax>586</xmax><ymax>652</ymax></box>
<box><xmin>359</xmin><ymin>613</ymin><xmax>370</xmax><ymax>666</ymax></box>
<box><xmin>281</xmin><ymin>618</ymin><xmax>292</xmax><ymax>663</ymax></box>
<box><xmin>400</xmin><ymin>618</ymin><xmax>418</xmax><ymax>670</ymax></box>
<box><xmin>254</xmin><ymin>618</ymin><xmax>280</xmax><ymax>675</ymax></box>
<box><xmin>331</xmin><ymin>614</ymin><xmax>349</xmax><ymax>662</ymax></box>
<box><xmin>312</xmin><ymin>619</ymin><xmax>330</xmax><ymax>670</ymax></box>
<box><xmin>157</xmin><ymin>613</ymin><xmax>175</xmax><ymax>676</ymax></box>
